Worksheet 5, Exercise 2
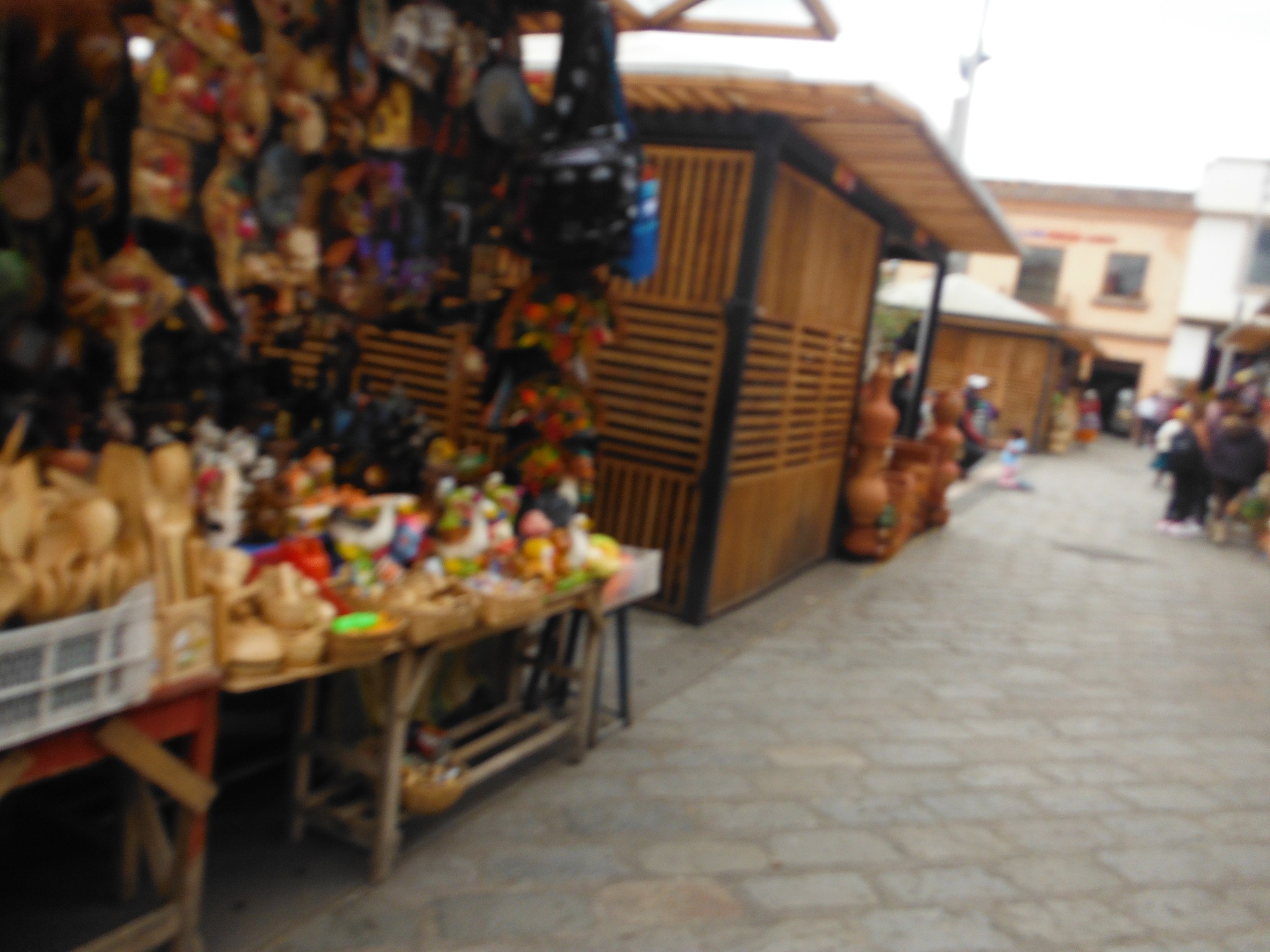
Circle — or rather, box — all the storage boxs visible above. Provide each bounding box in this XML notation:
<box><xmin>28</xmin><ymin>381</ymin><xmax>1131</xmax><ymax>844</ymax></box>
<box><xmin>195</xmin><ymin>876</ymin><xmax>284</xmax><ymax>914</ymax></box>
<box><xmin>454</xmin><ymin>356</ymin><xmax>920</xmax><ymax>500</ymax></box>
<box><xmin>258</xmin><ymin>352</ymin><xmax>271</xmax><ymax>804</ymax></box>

<box><xmin>0</xmin><ymin>573</ymin><xmax>224</xmax><ymax>750</ymax></box>
<box><xmin>598</xmin><ymin>541</ymin><xmax>665</xmax><ymax>616</ymax></box>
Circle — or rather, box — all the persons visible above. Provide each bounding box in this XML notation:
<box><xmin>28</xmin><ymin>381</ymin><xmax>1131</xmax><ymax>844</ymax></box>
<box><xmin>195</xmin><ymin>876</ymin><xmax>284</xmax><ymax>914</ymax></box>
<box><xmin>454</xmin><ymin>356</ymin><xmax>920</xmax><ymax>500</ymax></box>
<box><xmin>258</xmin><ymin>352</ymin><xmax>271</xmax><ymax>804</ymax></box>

<box><xmin>1079</xmin><ymin>375</ymin><xmax>1270</xmax><ymax>539</ymax></box>
<box><xmin>891</xmin><ymin>355</ymin><xmax>1029</xmax><ymax>491</ymax></box>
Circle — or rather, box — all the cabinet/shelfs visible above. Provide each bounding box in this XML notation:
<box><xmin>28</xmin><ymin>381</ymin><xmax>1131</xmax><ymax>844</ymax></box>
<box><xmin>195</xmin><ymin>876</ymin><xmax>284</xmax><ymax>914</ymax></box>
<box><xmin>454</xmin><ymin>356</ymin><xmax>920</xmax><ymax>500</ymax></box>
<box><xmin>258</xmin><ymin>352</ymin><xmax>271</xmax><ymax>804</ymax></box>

<box><xmin>226</xmin><ymin>535</ymin><xmax>630</xmax><ymax>877</ymax></box>
<box><xmin>0</xmin><ymin>671</ymin><xmax>210</xmax><ymax>952</ymax></box>
